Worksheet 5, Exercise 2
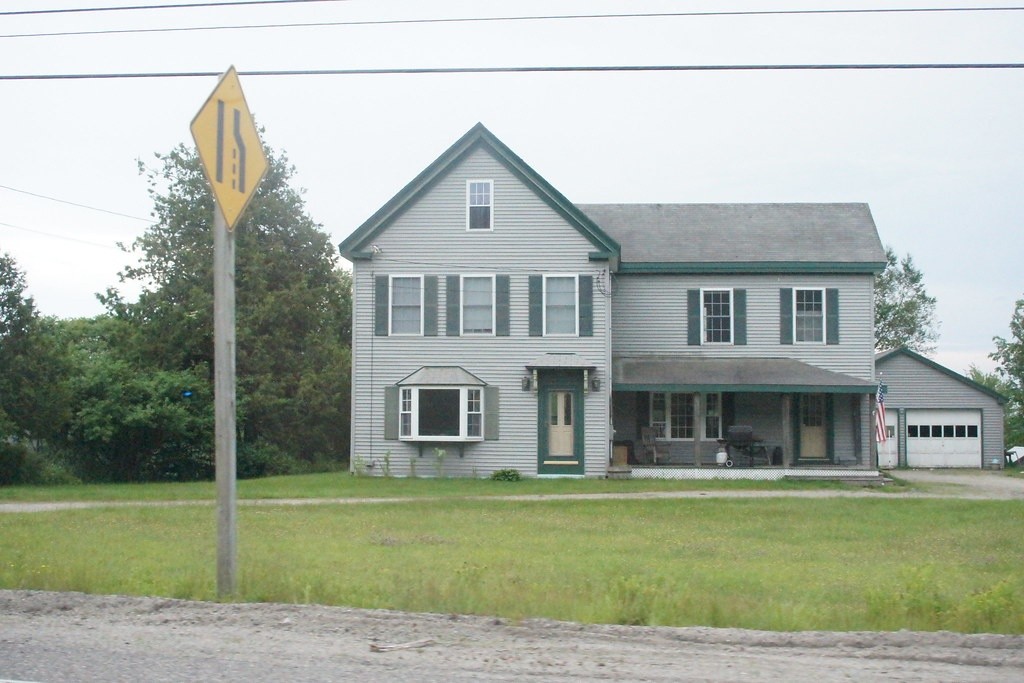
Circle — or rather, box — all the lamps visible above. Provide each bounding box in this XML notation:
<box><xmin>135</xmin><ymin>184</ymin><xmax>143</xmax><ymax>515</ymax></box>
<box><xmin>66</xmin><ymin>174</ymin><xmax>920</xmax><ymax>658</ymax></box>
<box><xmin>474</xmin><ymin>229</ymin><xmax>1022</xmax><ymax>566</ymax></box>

<box><xmin>522</xmin><ymin>377</ymin><xmax>530</xmax><ymax>391</ymax></box>
<box><xmin>592</xmin><ymin>377</ymin><xmax>600</xmax><ymax>392</ymax></box>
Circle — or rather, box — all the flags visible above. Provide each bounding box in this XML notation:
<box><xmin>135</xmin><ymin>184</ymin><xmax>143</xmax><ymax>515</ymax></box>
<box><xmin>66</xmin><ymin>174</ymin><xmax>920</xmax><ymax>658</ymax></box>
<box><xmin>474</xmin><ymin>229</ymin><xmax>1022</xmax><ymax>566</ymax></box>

<box><xmin>873</xmin><ymin>378</ymin><xmax>887</xmax><ymax>443</ymax></box>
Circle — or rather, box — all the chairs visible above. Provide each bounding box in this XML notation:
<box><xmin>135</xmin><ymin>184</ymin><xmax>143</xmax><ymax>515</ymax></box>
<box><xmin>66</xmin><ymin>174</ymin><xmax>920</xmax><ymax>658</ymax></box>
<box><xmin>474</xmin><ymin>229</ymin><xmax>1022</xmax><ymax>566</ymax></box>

<box><xmin>725</xmin><ymin>425</ymin><xmax>771</xmax><ymax>467</ymax></box>
<box><xmin>1004</xmin><ymin>447</ymin><xmax>1021</xmax><ymax>468</ymax></box>
<box><xmin>641</xmin><ymin>426</ymin><xmax>672</xmax><ymax>465</ymax></box>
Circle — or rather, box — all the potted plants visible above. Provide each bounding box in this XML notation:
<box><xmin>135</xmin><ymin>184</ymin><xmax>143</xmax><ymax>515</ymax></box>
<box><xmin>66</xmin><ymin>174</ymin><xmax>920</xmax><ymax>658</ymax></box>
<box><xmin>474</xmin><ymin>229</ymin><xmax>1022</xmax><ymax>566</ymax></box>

<box><xmin>990</xmin><ymin>458</ymin><xmax>1000</xmax><ymax>471</ymax></box>
